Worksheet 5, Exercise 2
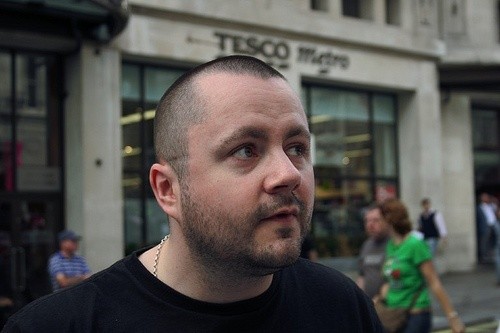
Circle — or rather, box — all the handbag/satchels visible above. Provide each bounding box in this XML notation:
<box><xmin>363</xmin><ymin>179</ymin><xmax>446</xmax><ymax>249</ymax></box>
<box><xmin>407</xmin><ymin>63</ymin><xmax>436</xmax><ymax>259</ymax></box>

<box><xmin>373</xmin><ymin>299</ymin><xmax>411</xmax><ymax>333</ymax></box>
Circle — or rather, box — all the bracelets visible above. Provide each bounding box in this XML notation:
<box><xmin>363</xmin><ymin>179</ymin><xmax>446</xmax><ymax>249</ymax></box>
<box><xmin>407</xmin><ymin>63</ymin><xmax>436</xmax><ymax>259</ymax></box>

<box><xmin>448</xmin><ymin>312</ymin><xmax>458</xmax><ymax>319</ymax></box>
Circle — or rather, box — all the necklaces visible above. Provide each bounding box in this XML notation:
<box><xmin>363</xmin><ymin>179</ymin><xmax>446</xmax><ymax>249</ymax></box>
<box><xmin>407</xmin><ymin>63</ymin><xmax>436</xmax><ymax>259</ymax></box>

<box><xmin>153</xmin><ymin>234</ymin><xmax>168</xmax><ymax>278</ymax></box>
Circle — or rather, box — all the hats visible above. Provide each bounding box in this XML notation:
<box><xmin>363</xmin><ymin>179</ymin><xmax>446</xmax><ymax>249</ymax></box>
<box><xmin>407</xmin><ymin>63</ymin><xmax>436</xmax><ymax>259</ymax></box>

<box><xmin>58</xmin><ymin>229</ymin><xmax>82</xmax><ymax>241</ymax></box>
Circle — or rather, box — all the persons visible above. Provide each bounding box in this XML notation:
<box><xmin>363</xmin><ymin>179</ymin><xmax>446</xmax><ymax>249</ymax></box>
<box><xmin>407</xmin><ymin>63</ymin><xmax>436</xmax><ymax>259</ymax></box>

<box><xmin>417</xmin><ymin>199</ymin><xmax>448</xmax><ymax>264</ymax></box>
<box><xmin>0</xmin><ymin>55</ymin><xmax>385</xmax><ymax>333</ymax></box>
<box><xmin>378</xmin><ymin>199</ymin><xmax>465</xmax><ymax>333</ymax></box>
<box><xmin>478</xmin><ymin>194</ymin><xmax>500</xmax><ymax>264</ymax></box>
<box><xmin>49</xmin><ymin>229</ymin><xmax>91</xmax><ymax>292</ymax></box>
<box><xmin>357</xmin><ymin>202</ymin><xmax>394</xmax><ymax>299</ymax></box>
